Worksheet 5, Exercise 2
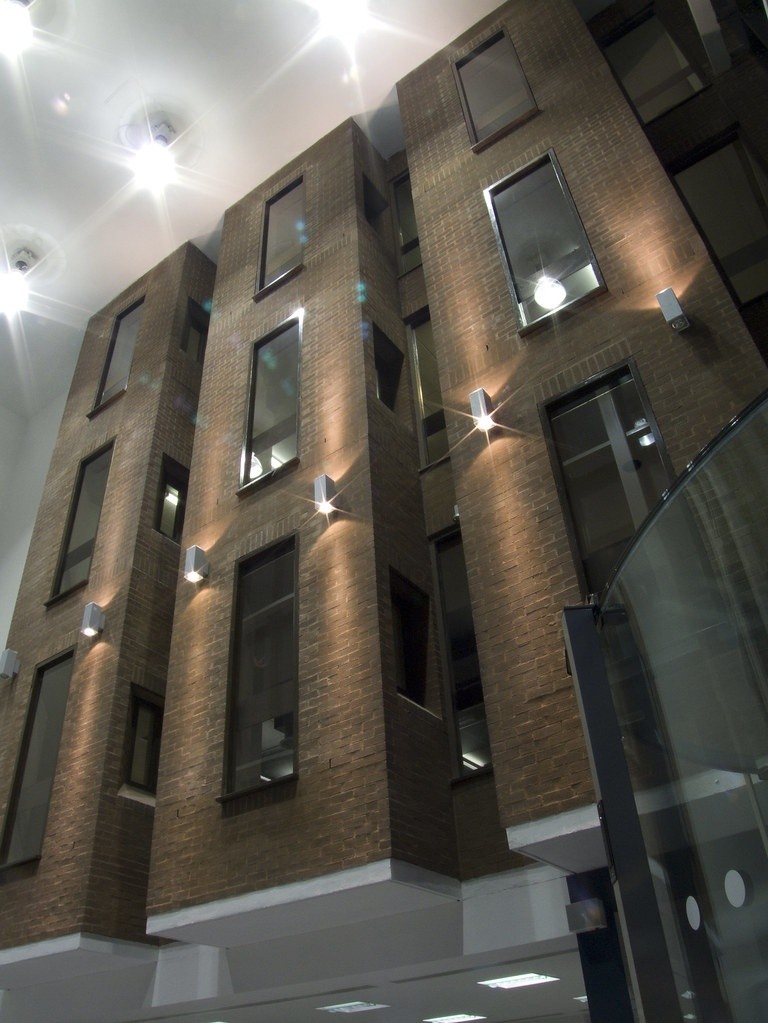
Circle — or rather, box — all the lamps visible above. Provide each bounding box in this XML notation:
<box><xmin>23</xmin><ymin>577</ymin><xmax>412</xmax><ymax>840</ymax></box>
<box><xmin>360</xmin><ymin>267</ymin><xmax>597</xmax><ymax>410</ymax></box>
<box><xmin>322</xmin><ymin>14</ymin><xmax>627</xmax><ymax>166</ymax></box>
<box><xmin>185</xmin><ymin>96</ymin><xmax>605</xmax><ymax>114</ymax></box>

<box><xmin>80</xmin><ymin>601</ymin><xmax>105</xmax><ymax>637</ymax></box>
<box><xmin>468</xmin><ymin>389</ymin><xmax>495</xmax><ymax>432</ymax></box>
<box><xmin>635</xmin><ymin>418</ymin><xmax>655</xmax><ymax>447</ymax></box>
<box><xmin>315</xmin><ymin>474</ymin><xmax>339</xmax><ymax>513</ymax></box>
<box><xmin>656</xmin><ymin>287</ymin><xmax>691</xmax><ymax>335</ymax></box>
<box><xmin>185</xmin><ymin>545</ymin><xmax>209</xmax><ymax>583</ymax></box>
<box><xmin>251</xmin><ymin>452</ymin><xmax>263</xmax><ymax>479</ymax></box>
<box><xmin>0</xmin><ymin>648</ymin><xmax>21</xmax><ymax>680</ymax></box>
<box><xmin>533</xmin><ymin>245</ymin><xmax>566</xmax><ymax>310</ymax></box>
<box><xmin>566</xmin><ymin>898</ymin><xmax>606</xmax><ymax>938</ymax></box>
